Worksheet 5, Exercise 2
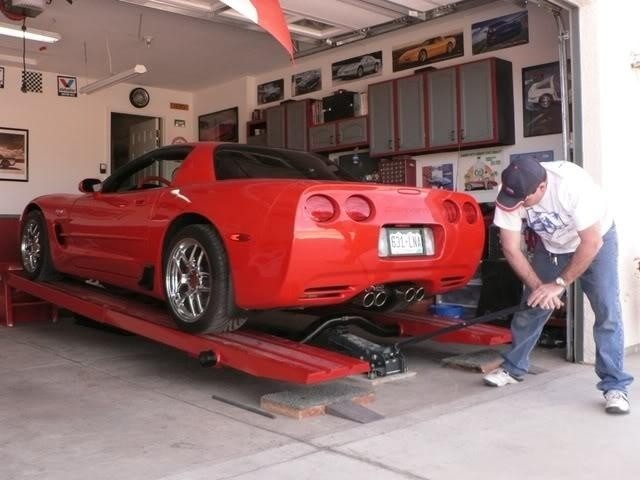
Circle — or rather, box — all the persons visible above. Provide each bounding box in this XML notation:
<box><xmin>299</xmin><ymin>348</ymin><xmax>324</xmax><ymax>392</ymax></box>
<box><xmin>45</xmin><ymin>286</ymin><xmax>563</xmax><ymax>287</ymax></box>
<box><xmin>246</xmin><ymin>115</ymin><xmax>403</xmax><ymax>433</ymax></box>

<box><xmin>480</xmin><ymin>152</ymin><xmax>633</xmax><ymax>417</ymax></box>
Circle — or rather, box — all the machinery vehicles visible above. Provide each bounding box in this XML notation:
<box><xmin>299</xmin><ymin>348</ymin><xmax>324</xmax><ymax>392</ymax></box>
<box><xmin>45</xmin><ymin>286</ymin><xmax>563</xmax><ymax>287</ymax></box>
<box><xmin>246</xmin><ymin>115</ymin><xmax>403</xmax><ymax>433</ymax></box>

<box><xmin>479</xmin><ymin>19</ymin><xmax>522</xmax><ymax>45</ymax></box>
<box><xmin>0</xmin><ymin>146</ymin><xmax>16</xmax><ymax>168</ymax></box>
<box><xmin>427</xmin><ymin>175</ymin><xmax>451</xmax><ymax>187</ymax></box>
<box><xmin>528</xmin><ymin>71</ymin><xmax>572</xmax><ymax>109</ymax></box>
<box><xmin>261</xmin><ymin>83</ymin><xmax>281</xmax><ymax>103</ymax></box>
<box><xmin>398</xmin><ymin>35</ymin><xmax>456</xmax><ymax>65</ymax></box>
<box><xmin>295</xmin><ymin>71</ymin><xmax>320</xmax><ymax>90</ymax></box>
<box><xmin>17</xmin><ymin>141</ymin><xmax>487</xmax><ymax>337</ymax></box>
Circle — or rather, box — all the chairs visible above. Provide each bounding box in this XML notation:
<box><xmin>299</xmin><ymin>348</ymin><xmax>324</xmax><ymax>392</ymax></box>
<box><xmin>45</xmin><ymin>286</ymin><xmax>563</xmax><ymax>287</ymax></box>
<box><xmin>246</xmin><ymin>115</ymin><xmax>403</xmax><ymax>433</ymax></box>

<box><xmin>483</xmin><ymin>366</ymin><xmax>523</xmax><ymax>387</ymax></box>
<box><xmin>604</xmin><ymin>389</ymin><xmax>630</xmax><ymax>414</ymax></box>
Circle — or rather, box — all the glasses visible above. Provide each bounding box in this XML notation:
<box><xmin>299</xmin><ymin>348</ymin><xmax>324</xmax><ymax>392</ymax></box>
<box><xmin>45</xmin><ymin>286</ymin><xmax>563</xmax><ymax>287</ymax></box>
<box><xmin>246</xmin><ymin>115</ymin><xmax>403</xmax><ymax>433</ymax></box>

<box><xmin>0</xmin><ymin>21</ymin><xmax>63</xmax><ymax>44</ymax></box>
<box><xmin>77</xmin><ymin>38</ymin><xmax>149</xmax><ymax>97</ymax></box>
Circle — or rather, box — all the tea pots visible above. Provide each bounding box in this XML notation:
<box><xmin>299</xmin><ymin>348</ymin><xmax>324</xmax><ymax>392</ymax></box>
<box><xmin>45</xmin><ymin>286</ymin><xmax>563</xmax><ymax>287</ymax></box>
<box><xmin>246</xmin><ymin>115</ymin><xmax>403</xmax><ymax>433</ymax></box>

<box><xmin>199</xmin><ymin>106</ymin><xmax>239</xmax><ymax>143</ymax></box>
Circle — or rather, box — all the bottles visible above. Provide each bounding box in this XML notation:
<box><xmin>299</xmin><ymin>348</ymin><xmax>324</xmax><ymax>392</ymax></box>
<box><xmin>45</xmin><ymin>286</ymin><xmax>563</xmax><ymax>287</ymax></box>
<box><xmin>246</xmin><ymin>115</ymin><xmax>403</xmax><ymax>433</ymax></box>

<box><xmin>495</xmin><ymin>158</ymin><xmax>546</xmax><ymax>212</ymax></box>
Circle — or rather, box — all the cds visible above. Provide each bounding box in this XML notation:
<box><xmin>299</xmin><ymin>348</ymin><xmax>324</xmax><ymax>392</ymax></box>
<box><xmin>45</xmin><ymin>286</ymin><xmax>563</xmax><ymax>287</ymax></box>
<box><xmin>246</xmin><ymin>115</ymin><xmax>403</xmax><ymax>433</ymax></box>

<box><xmin>548</xmin><ymin>252</ymin><xmax>558</xmax><ymax>267</ymax></box>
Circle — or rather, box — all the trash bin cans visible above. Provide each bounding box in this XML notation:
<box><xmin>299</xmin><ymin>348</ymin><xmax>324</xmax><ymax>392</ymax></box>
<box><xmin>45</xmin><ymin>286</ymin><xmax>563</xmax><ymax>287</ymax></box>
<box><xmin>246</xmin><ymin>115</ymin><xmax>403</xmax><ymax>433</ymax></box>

<box><xmin>552</xmin><ymin>273</ymin><xmax>569</xmax><ymax>290</ymax></box>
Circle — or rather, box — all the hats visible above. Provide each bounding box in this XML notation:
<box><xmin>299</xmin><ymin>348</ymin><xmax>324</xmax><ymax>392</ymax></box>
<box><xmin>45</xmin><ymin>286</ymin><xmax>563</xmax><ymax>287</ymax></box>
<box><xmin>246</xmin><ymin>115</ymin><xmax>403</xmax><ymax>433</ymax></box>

<box><xmin>463</xmin><ymin>158</ymin><xmax>498</xmax><ymax>192</ymax></box>
<box><xmin>336</xmin><ymin>55</ymin><xmax>381</xmax><ymax>78</ymax></box>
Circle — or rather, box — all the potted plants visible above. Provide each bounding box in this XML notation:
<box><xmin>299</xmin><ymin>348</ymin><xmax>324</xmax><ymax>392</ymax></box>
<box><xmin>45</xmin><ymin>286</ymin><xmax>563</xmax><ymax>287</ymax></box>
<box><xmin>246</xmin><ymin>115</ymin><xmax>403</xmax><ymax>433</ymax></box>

<box><xmin>0</xmin><ymin>127</ymin><xmax>29</xmax><ymax>183</ymax></box>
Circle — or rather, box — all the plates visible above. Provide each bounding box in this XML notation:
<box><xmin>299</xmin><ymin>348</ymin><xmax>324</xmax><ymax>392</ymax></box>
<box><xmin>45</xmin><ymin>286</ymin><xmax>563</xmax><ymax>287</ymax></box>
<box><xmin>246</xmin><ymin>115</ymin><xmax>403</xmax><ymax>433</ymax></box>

<box><xmin>424</xmin><ymin>56</ymin><xmax>517</xmax><ymax>153</ymax></box>
<box><xmin>307</xmin><ymin>114</ymin><xmax>367</xmax><ymax>153</ymax></box>
<box><xmin>246</xmin><ymin>118</ymin><xmax>266</xmax><ymax>147</ymax></box>
<box><xmin>264</xmin><ymin>97</ymin><xmax>323</xmax><ymax>152</ymax></box>
<box><xmin>366</xmin><ymin>73</ymin><xmax>426</xmax><ymax>160</ymax></box>
